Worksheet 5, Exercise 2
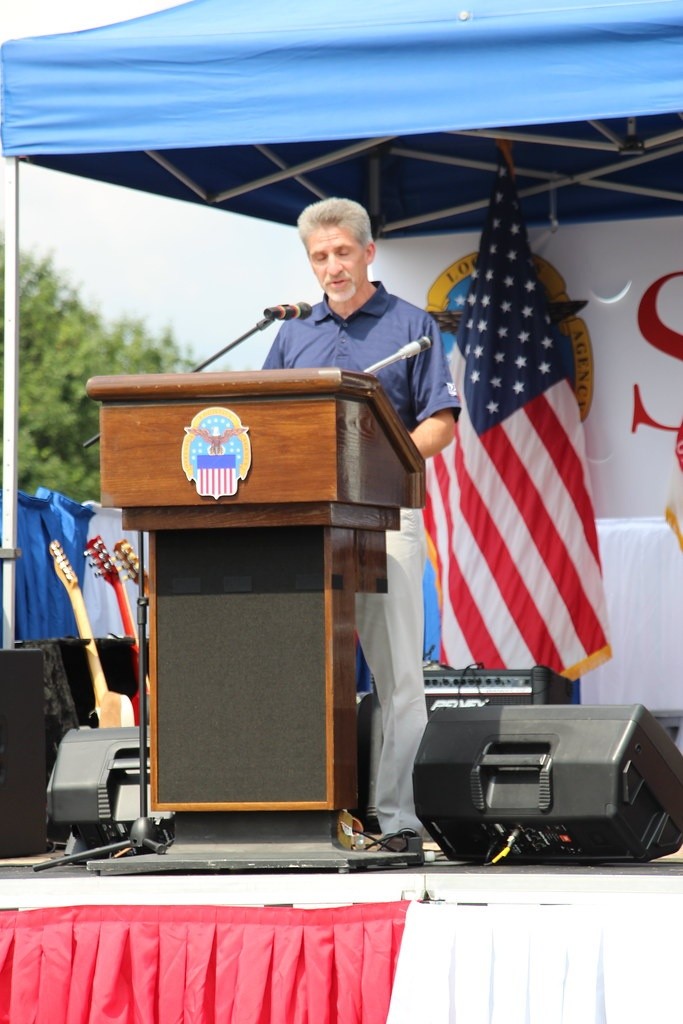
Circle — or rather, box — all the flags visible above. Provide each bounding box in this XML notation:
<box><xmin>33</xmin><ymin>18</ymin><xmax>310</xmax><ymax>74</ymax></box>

<box><xmin>434</xmin><ymin>156</ymin><xmax>612</xmax><ymax>683</ymax></box>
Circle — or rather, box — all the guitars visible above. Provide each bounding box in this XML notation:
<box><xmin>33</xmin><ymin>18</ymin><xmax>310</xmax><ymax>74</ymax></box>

<box><xmin>112</xmin><ymin>539</ymin><xmax>154</xmax><ymax>593</ymax></box>
<box><xmin>80</xmin><ymin>536</ymin><xmax>152</xmax><ymax>730</ymax></box>
<box><xmin>48</xmin><ymin>537</ymin><xmax>137</xmax><ymax>728</ymax></box>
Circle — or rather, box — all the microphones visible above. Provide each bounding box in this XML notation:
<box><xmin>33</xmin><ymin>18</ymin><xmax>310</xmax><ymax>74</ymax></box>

<box><xmin>264</xmin><ymin>302</ymin><xmax>312</xmax><ymax>320</ymax></box>
<box><xmin>364</xmin><ymin>336</ymin><xmax>433</xmax><ymax>376</ymax></box>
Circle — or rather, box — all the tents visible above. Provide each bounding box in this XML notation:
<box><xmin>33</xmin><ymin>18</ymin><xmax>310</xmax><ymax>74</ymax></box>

<box><xmin>0</xmin><ymin>0</ymin><xmax>683</xmax><ymax>650</ymax></box>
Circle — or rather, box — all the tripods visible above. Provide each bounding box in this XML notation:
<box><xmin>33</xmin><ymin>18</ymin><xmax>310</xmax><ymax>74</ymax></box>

<box><xmin>30</xmin><ymin>318</ymin><xmax>274</xmax><ymax>873</ymax></box>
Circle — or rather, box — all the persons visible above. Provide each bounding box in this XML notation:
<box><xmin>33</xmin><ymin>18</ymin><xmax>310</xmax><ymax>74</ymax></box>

<box><xmin>261</xmin><ymin>196</ymin><xmax>461</xmax><ymax>841</ymax></box>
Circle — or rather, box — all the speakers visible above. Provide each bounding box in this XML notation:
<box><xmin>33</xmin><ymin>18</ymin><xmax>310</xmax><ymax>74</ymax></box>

<box><xmin>412</xmin><ymin>703</ymin><xmax>682</xmax><ymax>865</ymax></box>
<box><xmin>43</xmin><ymin>724</ymin><xmax>173</xmax><ymax>860</ymax></box>
<box><xmin>422</xmin><ymin>665</ymin><xmax>572</xmax><ymax>719</ymax></box>
